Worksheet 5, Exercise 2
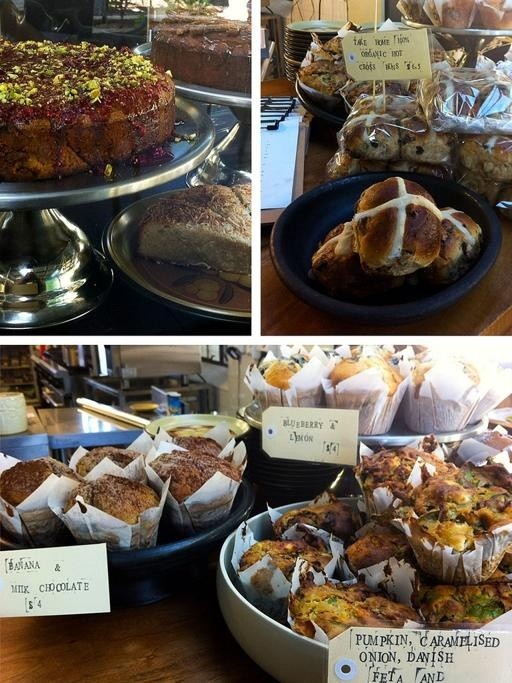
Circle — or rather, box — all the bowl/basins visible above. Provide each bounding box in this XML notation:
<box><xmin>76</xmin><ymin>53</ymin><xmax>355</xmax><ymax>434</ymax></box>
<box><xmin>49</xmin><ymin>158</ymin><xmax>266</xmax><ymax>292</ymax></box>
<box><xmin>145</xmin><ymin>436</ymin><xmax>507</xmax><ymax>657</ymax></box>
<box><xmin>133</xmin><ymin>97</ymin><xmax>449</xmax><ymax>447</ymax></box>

<box><xmin>215</xmin><ymin>496</ymin><xmax>364</xmax><ymax>682</ymax></box>
<box><xmin>1</xmin><ymin>479</ymin><xmax>258</xmax><ymax>609</ymax></box>
<box><xmin>271</xmin><ymin>174</ymin><xmax>504</xmax><ymax>324</ymax></box>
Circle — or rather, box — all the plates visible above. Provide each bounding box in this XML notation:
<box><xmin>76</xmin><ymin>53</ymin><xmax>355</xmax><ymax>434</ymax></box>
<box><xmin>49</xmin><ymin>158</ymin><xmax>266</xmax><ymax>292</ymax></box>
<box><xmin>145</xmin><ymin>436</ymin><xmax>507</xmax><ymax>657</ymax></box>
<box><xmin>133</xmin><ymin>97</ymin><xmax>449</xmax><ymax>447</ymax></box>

<box><xmin>281</xmin><ymin>21</ymin><xmax>348</xmax><ymax>87</ymax></box>
<box><xmin>292</xmin><ymin>81</ymin><xmax>348</xmax><ymax>128</ymax></box>
<box><xmin>103</xmin><ymin>185</ymin><xmax>254</xmax><ymax>319</ymax></box>
<box><xmin>234</xmin><ymin>407</ymin><xmax>341</xmax><ymax>508</ymax></box>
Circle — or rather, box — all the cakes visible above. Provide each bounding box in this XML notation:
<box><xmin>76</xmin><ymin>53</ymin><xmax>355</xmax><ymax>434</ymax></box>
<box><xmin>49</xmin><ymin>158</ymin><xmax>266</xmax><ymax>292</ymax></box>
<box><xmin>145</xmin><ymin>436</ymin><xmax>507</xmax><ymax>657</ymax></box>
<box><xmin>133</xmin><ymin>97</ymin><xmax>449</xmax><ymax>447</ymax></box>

<box><xmin>244</xmin><ymin>344</ymin><xmax>509</xmax><ymax>432</ymax></box>
<box><xmin>1</xmin><ymin>459</ymin><xmax>78</xmax><ymax>539</ymax></box>
<box><xmin>46</xmin><ymin>458</ymin><xmax>168</xmax><ymax>549</ymax></box>
<box><xmin>233</xmin><ymin>434</ymin><xmax>512</xmax><ymax>641</ymax></box>
<box><xmin>0</xmin><ymin>41</ymin><xmax>176</xmax><ymax>179</ymax></box>
<box><xmin>73</xmin><ymin>448</ymin><xmax>147</xmax><ymax>485</ymax></box>
<box><xmin>151</xmin><ymin>15</ymin><xmax>251</xmax><ymax>94</ymax></box>
<box><xmin>309</xmin><ymin>178</ymin><xmax>482</xmax><ymax>289</ymax></box>
<box><xmin>147</xmin><ymin>436</ymin><xmax>245</xmax><ymax>531</ymax></box>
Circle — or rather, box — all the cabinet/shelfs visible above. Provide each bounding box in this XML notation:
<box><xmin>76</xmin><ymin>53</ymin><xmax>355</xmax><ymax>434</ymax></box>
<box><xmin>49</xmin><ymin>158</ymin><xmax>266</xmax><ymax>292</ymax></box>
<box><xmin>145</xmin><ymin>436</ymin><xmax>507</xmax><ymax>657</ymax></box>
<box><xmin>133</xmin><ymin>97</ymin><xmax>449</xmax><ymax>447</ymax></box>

<box><xmin>1</xmin><ymin>345</ymin><xmax>44</xmax><ymax>409</ymax></box>
<box><xmin>85</xmin><ymin>378</ymin><xmax>217</xmax><ymax>415</ymax></box>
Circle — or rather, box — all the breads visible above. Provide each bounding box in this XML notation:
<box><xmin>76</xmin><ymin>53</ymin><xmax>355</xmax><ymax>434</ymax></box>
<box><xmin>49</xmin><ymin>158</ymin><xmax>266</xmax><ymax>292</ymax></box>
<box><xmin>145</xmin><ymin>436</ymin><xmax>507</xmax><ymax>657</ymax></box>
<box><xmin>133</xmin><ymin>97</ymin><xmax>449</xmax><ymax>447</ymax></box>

<box><xmin>137</xmin><ymin>183</ymin><xmax>252</xmax><ymax>292</ymax></box>
<box><xmin>297</xmin><ymin>1</ymin><xmax>512</xmax><ymax>177</ymax></box>
